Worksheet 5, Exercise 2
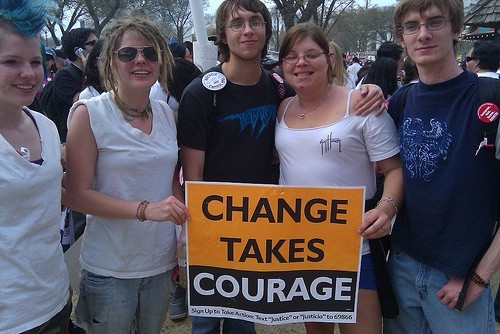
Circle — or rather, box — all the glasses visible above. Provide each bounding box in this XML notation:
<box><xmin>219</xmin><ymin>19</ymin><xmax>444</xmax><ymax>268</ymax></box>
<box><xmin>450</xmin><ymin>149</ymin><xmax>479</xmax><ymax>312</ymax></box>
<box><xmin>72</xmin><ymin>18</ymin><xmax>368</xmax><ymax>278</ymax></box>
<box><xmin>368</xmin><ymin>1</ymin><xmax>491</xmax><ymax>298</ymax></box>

<box><xmin>465</xmin><ymin>55</ymin><xmax>479</xmax><ymax>62</ymax></box>
<box><xmin>282</xmin><ymin>51</ymin><xmax>328</xmax><ymax>64</ymax></box>
<box><xmin>398</xmin><ymin>18</ymin><xmax>452</xmax><ymax>35</ymax></box>
<box><xmin>79</xmin><ymin>40</ymin><xmax>97</xmax><ymax>46</ymax></box>
<box><xmin>224</xmin><ymin>20</ymin><xmax>267</xmax><ymax>32</ymax></box>
<box><xmin>113</xmin><ymin>45</ymin><xmax>158</xmax><ymax>63</ymax></box>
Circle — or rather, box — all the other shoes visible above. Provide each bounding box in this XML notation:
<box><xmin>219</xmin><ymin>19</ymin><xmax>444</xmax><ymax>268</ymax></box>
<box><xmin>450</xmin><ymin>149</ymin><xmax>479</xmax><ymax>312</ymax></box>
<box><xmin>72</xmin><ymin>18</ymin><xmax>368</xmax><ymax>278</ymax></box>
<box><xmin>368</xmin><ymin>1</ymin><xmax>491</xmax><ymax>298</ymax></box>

<box><xmin>168</xmin><ymin>285</ymin><xmax>186</xmax><ymax>319</ymax></box>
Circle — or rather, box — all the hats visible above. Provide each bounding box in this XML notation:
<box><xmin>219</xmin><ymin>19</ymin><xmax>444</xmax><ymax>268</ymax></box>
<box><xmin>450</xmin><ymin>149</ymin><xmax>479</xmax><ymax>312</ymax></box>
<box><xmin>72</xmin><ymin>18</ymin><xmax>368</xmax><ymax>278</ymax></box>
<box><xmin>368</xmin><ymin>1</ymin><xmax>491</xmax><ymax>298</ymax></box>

<box><xmin>45</xmin><ymin>47</ymin><xmax>56</xmax><ymax>59</ymax></box>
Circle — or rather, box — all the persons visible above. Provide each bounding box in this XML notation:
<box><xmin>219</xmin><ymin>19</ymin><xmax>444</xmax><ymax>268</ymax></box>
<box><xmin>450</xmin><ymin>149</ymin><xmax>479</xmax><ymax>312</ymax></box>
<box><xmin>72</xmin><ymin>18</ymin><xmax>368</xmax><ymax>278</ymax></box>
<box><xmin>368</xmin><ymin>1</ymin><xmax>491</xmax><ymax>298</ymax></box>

<box><xmin>458</xmin><ymin>38</ymin><xmax>500</xmax><ymax>79</ymax></box>
<box><xmin>176</xmin><ymin>0</ymin><xmax>385</xmax><ymax>334</ymax></box>
<box><xmin>326</xmin><ymin>33</ymin><xmax>421</xmax><ymax>110</ymax></box>
<box><xmin>66</xmin><ymin>20</ymin><xmax>191</xmax><ymax>334</ymax></box>
<box><xmin>378</xmin><ymin>0</ymin><xmax>500</xmax><ymax>334</ymax></box>
<box><xmin>0</xmin><ymin>1</ymin><xmax>86</xmax><ymax>334</ymax></box>
<box><xmin>33</xmin><ymin>26</ymin><xmax>105</xmax><ymax>254</ymax></box>
<box><xmin>147</xmin><ymin>29</ymin><xmax>226</xmax><ymax>109</ymax></box>
<box><xmin>275</xmin><ymin>22</ymin><xmax>401</xmax><ymax>334</ymax></box>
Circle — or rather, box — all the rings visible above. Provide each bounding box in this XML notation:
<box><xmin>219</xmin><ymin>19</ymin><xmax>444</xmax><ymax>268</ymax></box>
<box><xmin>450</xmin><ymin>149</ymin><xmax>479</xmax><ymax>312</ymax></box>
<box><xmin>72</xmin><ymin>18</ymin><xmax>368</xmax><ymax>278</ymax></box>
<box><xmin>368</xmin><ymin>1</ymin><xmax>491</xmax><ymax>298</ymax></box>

<box><xmin>382</xmin><ymin>226</ymin><xmax>388</xmax><ymax>232</ymax></box>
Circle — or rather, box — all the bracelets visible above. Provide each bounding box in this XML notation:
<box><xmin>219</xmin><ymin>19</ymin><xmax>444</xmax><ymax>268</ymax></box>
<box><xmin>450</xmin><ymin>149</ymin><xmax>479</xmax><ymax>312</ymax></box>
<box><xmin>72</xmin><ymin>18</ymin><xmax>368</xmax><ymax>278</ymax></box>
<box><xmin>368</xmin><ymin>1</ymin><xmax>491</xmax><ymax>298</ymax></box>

<box><xmin>471</xmin><ymin>271</ymin><xmax>489</xmax><ymax>288</ymax></box>
<box><xmin>137</xmin><ymin>200</ymin><xmax>150</xmax><ymax>222</ymax></box>
<box><xmin>377</xmin><ymin>197</ymin><xmax>398</xmax><ymax>217</ymax></box>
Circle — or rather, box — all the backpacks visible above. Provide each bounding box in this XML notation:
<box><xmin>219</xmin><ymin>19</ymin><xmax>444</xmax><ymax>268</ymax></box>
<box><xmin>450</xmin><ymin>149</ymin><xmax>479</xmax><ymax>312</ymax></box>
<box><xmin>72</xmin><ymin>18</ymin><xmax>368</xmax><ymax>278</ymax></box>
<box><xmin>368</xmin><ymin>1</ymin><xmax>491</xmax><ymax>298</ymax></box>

<box><xmin>38</xmin><ymin>81</ymin><xmax>60</xmax><ymax>127</ymax></box>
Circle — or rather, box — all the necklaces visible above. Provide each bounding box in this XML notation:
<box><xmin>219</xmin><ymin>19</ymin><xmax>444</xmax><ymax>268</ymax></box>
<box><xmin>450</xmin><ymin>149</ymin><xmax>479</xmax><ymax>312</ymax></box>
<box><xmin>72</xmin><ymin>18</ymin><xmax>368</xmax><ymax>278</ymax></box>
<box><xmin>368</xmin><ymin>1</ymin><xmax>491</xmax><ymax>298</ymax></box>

<box><xmin>298</xmin><ymin>92</ymin><xmax>330</xmax><ymax>119</ymax></box>
<box><xmin>4</xmin><ymin>113</ymin><xmax>35</xmax><ymax>155</ymax></box>
